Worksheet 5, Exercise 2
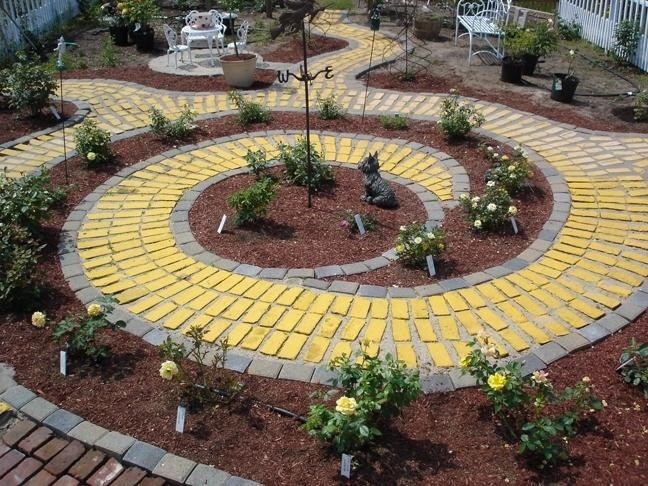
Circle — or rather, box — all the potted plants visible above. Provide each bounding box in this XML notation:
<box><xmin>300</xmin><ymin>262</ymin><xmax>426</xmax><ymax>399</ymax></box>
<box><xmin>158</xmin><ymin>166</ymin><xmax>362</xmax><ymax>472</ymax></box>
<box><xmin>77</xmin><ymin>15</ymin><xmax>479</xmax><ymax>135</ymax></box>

<box><xmin>519</xmin><ymin>15</ymin><xmax>559</xmax><ymax>75</ymax></box>
<box><xmin>413</xmin><ymin>11</ymin><xmax>443</xmax><ymax>39</ymax></box>
<box><xmin>216</xmin><ymin>0</ymin><xmax>257</xmax><ymax>88</ymax></box>
<box><xmin>497</xmin><ymin>21</ymin><xmax>535</xmax><ymax>83</ymax></box>
<box><xmin>214</xmin><ymin>0</ymin><xmax>239</xmax><ymax>36</ymax></box>
<box><xmin>551</xmin><ymin>48</ymin><xmax>580</xmax><ymax>103</ymax></box>
<box><xmin>99</xmin><ymin>2</ymin><xmax>129</xmax><ymax>45</ymax></box>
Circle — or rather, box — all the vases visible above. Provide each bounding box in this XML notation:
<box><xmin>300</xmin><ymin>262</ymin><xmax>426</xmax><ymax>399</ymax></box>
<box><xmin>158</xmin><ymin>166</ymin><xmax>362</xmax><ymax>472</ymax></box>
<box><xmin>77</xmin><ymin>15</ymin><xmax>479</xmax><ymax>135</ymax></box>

<box><xmin>131</xmin><ymin>25</ymin><xmax>155</xmax><ymax>52</ymax></box>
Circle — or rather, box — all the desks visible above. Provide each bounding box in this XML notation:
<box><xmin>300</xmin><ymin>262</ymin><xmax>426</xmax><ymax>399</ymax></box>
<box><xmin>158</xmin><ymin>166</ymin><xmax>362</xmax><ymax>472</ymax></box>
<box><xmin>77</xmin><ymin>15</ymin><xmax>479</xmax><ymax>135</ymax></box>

<box><xmin>182</xmin><ymin>26</ymin><xmax>223</xmax><ymax>66</ymax></box>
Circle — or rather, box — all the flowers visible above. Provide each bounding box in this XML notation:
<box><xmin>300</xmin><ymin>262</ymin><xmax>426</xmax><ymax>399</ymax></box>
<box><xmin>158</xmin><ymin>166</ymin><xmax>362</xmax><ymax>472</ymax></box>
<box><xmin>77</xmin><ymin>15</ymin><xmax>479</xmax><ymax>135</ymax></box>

<box><xmin>116</xmin><ymin>0</ymin><xmax>162</xmax><ymax>29</ymax></box>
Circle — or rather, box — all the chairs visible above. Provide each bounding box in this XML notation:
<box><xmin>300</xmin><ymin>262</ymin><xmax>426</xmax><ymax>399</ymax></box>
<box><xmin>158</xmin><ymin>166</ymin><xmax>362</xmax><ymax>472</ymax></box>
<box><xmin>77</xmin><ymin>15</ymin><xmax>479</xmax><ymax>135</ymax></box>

<box><xmin>163</xmin><ymin>23</ymin><xmax>192</xmax><ymax>68</ymax></box>
<box><xmin>227</xmin><ymin>19</ymin><xmax>249</xmax><ymax>55</ymax></box>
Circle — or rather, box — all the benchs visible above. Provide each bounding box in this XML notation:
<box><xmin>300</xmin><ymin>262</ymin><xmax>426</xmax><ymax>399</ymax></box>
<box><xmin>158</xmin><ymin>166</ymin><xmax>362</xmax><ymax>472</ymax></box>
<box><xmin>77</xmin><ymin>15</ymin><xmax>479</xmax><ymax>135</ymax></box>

<box><xmin>455</xmin><ymin>0</ymin><xmax>512</xmax><ymax>66</ymax></box>
<box><xmin>185</xmin><ymin>9</ymin><xmax>227</xmax><ymax>59</ymax></box>
